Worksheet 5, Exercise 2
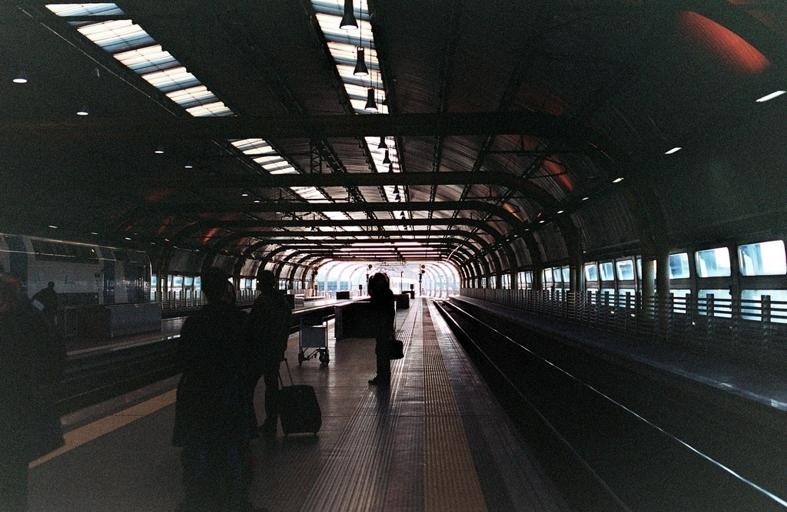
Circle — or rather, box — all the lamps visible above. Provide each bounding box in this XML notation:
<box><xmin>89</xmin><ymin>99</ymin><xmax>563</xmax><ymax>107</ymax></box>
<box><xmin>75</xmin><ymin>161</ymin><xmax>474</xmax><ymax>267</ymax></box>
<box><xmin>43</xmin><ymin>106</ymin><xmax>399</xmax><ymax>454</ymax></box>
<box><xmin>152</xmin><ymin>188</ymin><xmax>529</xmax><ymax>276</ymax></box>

<box><xmin>337</xmin><ymin>0</ymin><xmax>409</xmax><ymax>238</ymax></box>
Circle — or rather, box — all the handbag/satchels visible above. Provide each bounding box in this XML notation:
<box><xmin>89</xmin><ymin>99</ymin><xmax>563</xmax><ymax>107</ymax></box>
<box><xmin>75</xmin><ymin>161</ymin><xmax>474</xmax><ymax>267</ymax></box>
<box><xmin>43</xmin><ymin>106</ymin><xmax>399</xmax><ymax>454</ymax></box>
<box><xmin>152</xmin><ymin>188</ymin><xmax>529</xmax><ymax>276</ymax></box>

<box><xmin>390</xmin><ymin>335</ymin><xmax>404</xmax><ymax>359</ymax></box>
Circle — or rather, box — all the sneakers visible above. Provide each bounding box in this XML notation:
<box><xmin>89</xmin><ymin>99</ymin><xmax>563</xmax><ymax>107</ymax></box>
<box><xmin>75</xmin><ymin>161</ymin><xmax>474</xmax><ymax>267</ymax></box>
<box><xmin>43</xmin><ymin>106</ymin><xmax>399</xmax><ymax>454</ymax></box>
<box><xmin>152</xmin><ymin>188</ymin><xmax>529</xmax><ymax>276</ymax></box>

<box><xmin>368</xmin><ymin>376</ymin><xmax>390</xmax><ymax>385</ymax></box>
<box><xmin>258</xmin><ymin>424</ymin><xmax>277</xmax><ymax>434</ymax></box>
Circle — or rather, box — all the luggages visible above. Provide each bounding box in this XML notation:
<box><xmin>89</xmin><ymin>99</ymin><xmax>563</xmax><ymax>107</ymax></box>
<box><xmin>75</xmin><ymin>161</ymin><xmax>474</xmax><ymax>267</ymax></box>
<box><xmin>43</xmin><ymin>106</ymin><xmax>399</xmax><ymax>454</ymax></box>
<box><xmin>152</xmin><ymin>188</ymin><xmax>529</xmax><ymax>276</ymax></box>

<box><xmin>272</xmin><ymin>358</ymin><xmax>322</xmax><ymax>438</ymax></box>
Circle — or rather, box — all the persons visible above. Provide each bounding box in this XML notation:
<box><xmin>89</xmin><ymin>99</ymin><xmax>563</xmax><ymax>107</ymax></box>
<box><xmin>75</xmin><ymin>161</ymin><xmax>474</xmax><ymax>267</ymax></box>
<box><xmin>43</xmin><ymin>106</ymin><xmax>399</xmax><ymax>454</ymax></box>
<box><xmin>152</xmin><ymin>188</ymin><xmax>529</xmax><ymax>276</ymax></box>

<box><xmin>368</xmin><ymin>273</ymin><xmax>395</xmax><ymax>386</ymax></box>
<box><xmin>0</xmin><ymin>272</ymin><xmax>65</xmax><ymax>512</ymax></box>
<box><xmin>172</xmin><ymin>265</ymin><xmax>266</xmax><ymax>512</ymax></box>
<box><xmin>224</xmin><ymin>281</ymin><xmax>236</xmax><ymax>304</ymax></box>
<box><xmin>248</xmin><ymin>270</ymin><xmax>292</xmax><ymax>432</ymax></box>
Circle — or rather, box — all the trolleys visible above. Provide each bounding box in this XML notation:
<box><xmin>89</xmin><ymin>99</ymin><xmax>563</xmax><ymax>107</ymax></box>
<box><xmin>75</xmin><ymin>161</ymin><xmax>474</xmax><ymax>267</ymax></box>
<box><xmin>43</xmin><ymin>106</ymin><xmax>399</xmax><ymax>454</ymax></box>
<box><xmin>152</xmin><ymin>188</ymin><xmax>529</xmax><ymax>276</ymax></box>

<box><xmin>295</xmin><ymin>316</ymin><xmax>333</xmax><ymax>369</ymax></box>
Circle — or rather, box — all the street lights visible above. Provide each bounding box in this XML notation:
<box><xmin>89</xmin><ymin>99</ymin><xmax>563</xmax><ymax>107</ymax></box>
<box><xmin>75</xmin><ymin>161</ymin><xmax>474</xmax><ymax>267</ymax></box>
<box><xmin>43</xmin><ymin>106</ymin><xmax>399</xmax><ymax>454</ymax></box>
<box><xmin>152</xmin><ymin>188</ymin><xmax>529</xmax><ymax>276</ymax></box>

<box><xmin>400</xmin><ymin>270</ymin><xmax>403</xmax><ymax>293</ymax></box>
